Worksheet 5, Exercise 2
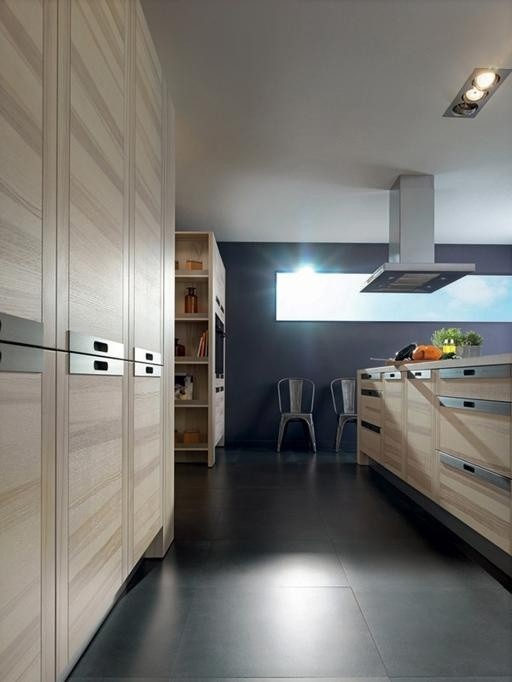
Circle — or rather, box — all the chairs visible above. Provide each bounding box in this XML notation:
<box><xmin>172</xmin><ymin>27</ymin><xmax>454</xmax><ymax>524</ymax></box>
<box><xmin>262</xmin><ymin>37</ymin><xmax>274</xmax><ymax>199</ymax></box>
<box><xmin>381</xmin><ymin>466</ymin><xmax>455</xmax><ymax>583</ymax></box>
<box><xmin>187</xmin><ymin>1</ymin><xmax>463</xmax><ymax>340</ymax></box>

<box><xmin>329</xmin><ymin>377</ymin><xmax>360</xmax><ymax>454</ymax></box>
<box><xmin>275</xmin><ymin>376</ymin><xmax>319</xmax><ymax>454</ymax></box>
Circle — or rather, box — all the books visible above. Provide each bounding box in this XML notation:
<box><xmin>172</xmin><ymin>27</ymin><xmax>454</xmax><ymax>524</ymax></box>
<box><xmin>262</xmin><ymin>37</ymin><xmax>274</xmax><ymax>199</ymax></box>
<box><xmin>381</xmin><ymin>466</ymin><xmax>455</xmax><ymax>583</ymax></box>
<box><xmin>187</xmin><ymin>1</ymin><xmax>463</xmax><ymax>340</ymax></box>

<box><xmin>196</xmin><ymin>330</ymin><xmax>208</xmax><ymax>357</ymax></box>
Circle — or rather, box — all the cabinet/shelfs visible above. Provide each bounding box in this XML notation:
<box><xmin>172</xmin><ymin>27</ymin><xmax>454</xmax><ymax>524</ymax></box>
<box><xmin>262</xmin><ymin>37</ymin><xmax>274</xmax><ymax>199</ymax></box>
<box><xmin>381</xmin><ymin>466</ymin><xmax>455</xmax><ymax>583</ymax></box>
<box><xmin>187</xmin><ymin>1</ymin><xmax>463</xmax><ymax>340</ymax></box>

<box><xmin>357</xmin><ymin>373</ymin><xmax>382</xmax><ymax>466</ymax></box>
<box><xmin>1</xmin><ymin>2</ymin><xmax>177</xmax><ymax>681</ymax></box>
<box><xmin>431</xmin><ymin>363</ymin><xmax>511</xmax><ymax>567</ymax></box>
<box><xmin>173</xmin><ymin>229</ymin><xmax>229</xmax><ymax>469</ymax></box>
<box><xmin>380</xmin><ymin>369</ymin><xmax>437</xmax><ymax>505</ymax></box>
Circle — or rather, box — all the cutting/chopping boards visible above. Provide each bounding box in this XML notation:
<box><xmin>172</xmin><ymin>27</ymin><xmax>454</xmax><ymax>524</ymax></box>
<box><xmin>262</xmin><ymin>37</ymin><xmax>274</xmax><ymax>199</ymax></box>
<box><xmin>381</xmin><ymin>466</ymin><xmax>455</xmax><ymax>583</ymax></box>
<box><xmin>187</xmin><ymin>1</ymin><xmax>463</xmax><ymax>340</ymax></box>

<box><xmin>384</xmin><ymin>359</ymin><xmax>438</xmax><ymax>366</ymax></box>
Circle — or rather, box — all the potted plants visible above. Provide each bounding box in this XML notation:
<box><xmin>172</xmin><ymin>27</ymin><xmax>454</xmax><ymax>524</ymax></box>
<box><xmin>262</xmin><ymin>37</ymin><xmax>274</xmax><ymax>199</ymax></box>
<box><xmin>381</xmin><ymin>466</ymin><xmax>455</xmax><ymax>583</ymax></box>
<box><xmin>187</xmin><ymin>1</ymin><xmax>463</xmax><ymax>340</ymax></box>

<box><xmin>430</xmin><ymin>327</ymin><xmax>484</xmax><ymax>361</ymax></box>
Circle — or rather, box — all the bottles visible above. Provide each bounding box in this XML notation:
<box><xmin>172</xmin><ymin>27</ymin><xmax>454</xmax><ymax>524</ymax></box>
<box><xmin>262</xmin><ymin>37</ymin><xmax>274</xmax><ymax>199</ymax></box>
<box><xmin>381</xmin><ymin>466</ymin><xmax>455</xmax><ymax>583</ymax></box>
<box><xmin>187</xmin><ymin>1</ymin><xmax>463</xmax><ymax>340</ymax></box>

<box><xmin>442</xmin><ymin>338</ymin><xmax>456</xmax><ymax>355</ymax></box>
<box><xmin>175</xmin><ymin>337</ymin><xmax>186</xmax><ymax>357</ymax></box>
<box><xmin>460</xmin><ymin>342</ymin><xmax>471</xmax><ymax>358</ymax></box>
<box><xmin>184</xmin><ymin>286</ymin><xmax>197</xmax><ymax>314</ymax></box>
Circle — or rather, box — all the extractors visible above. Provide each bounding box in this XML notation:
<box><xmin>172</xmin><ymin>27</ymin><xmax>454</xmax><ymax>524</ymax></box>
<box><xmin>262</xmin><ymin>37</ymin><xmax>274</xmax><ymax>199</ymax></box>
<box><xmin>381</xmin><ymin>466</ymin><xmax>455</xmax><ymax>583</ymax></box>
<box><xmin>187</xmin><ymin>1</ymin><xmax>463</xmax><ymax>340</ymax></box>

<box><xmin>359</xmin><ymin>172</ymin><xmax>477</xmax><ymax>295</ymax></box>
